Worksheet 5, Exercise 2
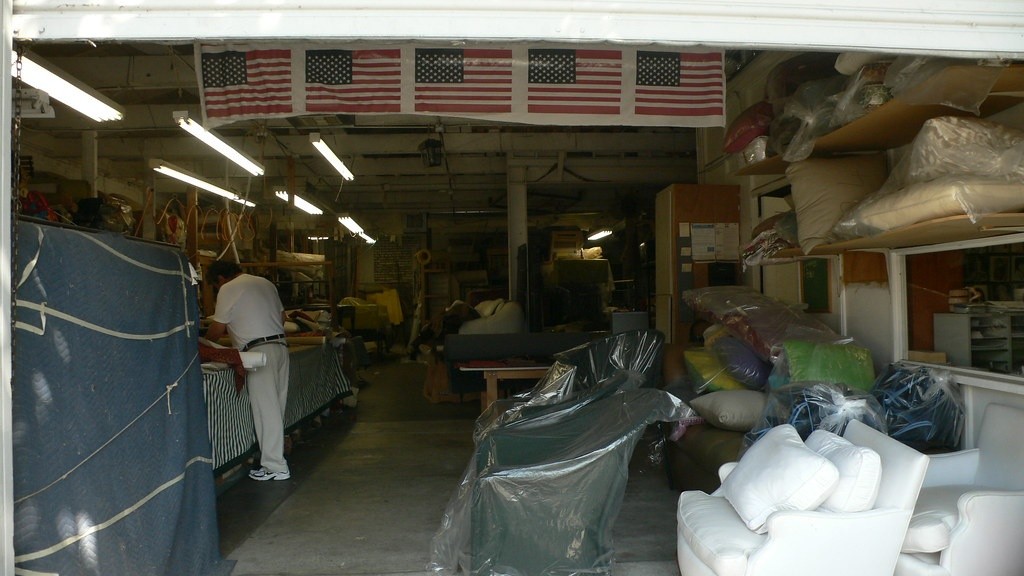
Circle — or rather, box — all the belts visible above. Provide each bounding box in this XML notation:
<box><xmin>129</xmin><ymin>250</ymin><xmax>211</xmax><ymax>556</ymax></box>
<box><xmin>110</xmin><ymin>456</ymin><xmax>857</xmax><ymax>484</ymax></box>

<box><xmin>243</xmin><ymin>334</ymin><xmax>285</xmax><ymax>351</ymax></box>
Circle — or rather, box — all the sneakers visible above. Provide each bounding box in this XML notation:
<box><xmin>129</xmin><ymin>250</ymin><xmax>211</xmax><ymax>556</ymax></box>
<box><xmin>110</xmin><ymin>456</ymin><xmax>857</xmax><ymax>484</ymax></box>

<box><xmin>248</xmin><ymin>465</ymin><xmax>291</xmax><ymax>481</ymax></box>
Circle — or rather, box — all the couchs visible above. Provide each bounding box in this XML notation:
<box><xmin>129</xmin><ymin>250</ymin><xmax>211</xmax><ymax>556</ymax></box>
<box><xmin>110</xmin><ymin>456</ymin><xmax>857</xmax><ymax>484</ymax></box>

<box><xmin>499</xmin><ymin>329</ymin><xmax>665</xmax><ymax>429</ymax></box>
<box><xmin>469</xmin><ymin>387</ymin><xmax>665</xmax><ymax>576</ymax></box>
<box><xmin>657</xmin><ymin>286</ymin><xmax>1024</xmax><ymax>576</ymax></box>
<box><xmin>410</xmin><ymin>298</ymin><xmax>524</xmax><ymax>403</ymax></box>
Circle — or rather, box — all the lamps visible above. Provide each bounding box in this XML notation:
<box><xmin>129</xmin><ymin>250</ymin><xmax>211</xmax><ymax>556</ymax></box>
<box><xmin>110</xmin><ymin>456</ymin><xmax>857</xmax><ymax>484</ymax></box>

<box><xmin>335</xmin><ymin>212</ymin><xmax>377</xmax><ymax>245</ymax></box>
<box><xmin>308</xmin><ymin>133</ymin><xmax>355</xmax><ymax>182</ymax></box>
<box><xmin>418</xmin><ymin>138</ymin><xmax>444</xmax><ymax>167</ymax></box>
<box><xmin>12</xmin><ymin>88</ymin><xmax>55</xmax><ymax>118</ymax></box>
<box><xmin>586</xmin><ymin>227</ymin><xmax>614</xmax><ymax>241</ymax></box>
<box><xmin>12</xmin><ymin>42</ymin><xmax>125</xmax><ymax>123</ymax></box>
<box><xmin>148</xmin><ymin>158</ymin><xmax>241</xmax><ymax>201</ymax></box>
<box><xmin>172</xmin><ymin>110</ymin><xmax>266</xmax><ymax>177</ymax></box>
<box><xmin>272</xmin><ymin>185</ymin><xmax>324</xmax><ymax>215</ymax></box>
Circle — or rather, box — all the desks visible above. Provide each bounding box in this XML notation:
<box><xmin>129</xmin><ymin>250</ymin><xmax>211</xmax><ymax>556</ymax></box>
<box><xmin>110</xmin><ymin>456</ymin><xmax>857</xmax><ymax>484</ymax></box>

<box><xmin>445</xmin><ymin>332</ymin><xmax>606</xmax><ymax>423</ymax></box>
<box><xmin>611</xmin><ymin>312</ymin><xmax>649</xmax><ymax>335</ymax></box>
<box><xmin>201</xmin><ymin>343</ymin><xmax>355</xmax><ymax>481</ymax></box>
<box><xmin>305</xmin><ymin>304</ymin><xmax>391</xmax><ymax>361</ymax></box>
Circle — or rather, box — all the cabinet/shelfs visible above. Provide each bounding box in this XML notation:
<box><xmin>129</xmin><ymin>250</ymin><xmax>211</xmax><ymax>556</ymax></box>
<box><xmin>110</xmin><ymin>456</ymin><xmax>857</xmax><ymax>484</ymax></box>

<box><xmin>722</xmin><ymin>48</ymin><xmax>1024</xmax><ymax>396</ymax></box>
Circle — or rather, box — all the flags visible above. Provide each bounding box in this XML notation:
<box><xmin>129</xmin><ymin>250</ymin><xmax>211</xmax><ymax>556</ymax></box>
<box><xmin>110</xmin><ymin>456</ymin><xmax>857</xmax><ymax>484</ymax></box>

<box><xmin>202</xmin><ymin>48</ymin><xmax>723</xmax><ymax>118</ymax></box>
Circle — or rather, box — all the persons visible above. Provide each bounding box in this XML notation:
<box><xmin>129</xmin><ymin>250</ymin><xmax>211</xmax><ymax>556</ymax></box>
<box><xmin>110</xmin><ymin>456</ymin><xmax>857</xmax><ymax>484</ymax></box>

<box><xmin>204</xmin><ymin>261</ymin><xmax>290</xmax><ymax>481</ymax></box>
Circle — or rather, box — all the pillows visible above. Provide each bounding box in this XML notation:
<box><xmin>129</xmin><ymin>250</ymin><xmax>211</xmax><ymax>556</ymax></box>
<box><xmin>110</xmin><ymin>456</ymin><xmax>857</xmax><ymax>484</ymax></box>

<box><xmin>762</xmin><ymin>52</ymin><xmax>840</xmax><ymax>101</ymax></box>
<box><xmin>881</xmin><ymin>54</ymin><xmax>939</xmax><ymax>88</ymax></box>
<box><xmin>689</xmin><ymin>390</ymin><xmax>789</xmax><ymax>430</ymax></box>
<box><xmin>784</xmin><ymin>152</ymin><xmax>888</xmax><ymax>256</ymax></box>
<box><xmin>827</xmin><ymin>83</ymin><xmax>891</xmax><ymax>128</ymax></box>
<box><xmin>903</xmin><ymin>115</ymin><xmax>1024</xmax><ymax>187</ymax></box>
<box><xmin>790</xmin><ymin>73</ymin><xmax>845</xmax><ymax>140</ymax></box>
<box><xmin>767</xmin><ymin>111</ymin><xmax>802</xmax><ymax>155</ymax></box>
<box><xmin>833</xmin><ymin>51</ymin><xmax>900</xmax><ymax>76</ymax></box>
<box><xmin>803</xmin><ymin>429</ymin><xmax>883</xmax><ymax>513</ymax></box>
<box><xmin>847</xmin><ymin>59</ymin><xmax>889</xmax><ymax>88</ymax></box>
<box><xmin>714</xmin><ymin>337</ymin><xmax>773</xmax><ymax>393</ymax></box>
<box><xmin>751</xmin><ymin>209</ymin><xmax>800</xmax><ymax>246</ymax></box>
<box><xmin>856</xmin><ymin>174</ymin><xmax>1024</xmax><ymax>231</ymax></box>
<box><xmin>723</xmin><ymin>101</ymin><xmax>773</xmax><ymax>154</ymax></box>
<box><xmin>890</xmin><ymin>58</ymin><xmax>964</xmax><ymax>98</ymax></box>
<box><xmin>683</xmin><ymin>351</ymin><xmax>750</xmax><ymax>393</ymax></box>
<box><xmin>737</xmin><ymin>135</ymin><xmax>768</xmax><ymax>168</ymax></box>
<box><xmin>721</xmin><ymin>424</ymin><xmax>840</xmax><ymax>536</ymax></box>
<box><xmin>782</xmin><ymin>340</ymin><xmax>875</xmax><ymax>399</ymax></box>
